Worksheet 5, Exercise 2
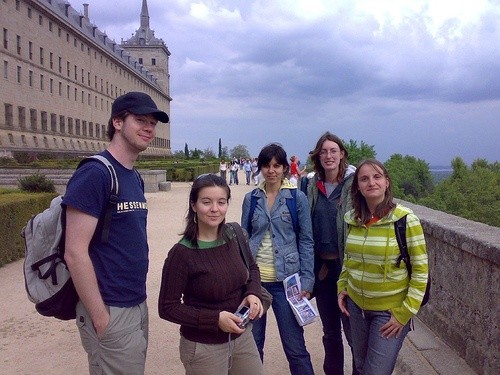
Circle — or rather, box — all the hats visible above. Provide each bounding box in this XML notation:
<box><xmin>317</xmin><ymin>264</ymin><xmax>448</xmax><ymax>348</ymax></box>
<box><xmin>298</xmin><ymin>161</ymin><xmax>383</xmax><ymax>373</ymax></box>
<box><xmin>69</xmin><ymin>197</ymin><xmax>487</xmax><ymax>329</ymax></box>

<box><xmin>112</xmin><ymin>92</ymin><xmax>170</xmax><ymax>124</ymax></box>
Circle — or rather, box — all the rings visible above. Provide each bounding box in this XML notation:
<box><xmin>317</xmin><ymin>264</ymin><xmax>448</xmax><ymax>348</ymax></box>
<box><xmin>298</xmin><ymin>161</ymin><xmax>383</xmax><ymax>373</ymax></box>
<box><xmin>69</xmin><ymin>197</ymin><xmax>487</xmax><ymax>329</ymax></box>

<box><xmin>254</xmin><ymin>302</ymin><xmax>258</xmax><ymax>306</ymax></box>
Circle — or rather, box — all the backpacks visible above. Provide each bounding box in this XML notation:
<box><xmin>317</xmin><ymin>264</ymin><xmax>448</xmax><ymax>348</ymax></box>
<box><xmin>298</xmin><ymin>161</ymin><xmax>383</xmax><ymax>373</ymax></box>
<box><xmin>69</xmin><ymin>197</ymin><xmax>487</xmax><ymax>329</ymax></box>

<box><xmin>21</xmin><ymin>155</ymin><xmax>142</xmax><ymax>321</ymax></box>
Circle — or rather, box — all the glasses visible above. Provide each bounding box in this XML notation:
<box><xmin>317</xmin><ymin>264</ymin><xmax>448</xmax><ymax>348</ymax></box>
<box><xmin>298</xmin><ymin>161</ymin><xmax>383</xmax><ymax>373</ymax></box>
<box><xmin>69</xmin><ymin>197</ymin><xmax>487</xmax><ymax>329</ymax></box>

<box><xmin>197</xmin><ymin>174</ymin><xmax>223</xmax><ymax>182</ymax></box>
<box><xmin>320</xmin><ymin>149</ymin><xmax>341</xmax><ymax>155</ymax></box>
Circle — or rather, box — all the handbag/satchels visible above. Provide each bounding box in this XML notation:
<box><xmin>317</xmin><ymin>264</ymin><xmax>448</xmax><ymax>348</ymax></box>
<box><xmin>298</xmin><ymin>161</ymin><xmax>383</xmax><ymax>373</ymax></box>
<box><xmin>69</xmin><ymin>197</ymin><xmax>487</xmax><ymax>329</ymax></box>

<box><xmin>226</xmin><ymin>222</ymin><xmax>273</xmax><ymax>324</ymax></box>
<box><xmin>394</xmin><ymin>213</ymin><xmax>431</xmax><ymax>306</ymax></box>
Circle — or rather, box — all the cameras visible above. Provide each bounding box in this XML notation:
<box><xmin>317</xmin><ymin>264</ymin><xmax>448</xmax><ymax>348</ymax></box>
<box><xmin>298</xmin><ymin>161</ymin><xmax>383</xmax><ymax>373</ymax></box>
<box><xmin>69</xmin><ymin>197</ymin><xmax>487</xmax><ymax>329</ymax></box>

<box><xmin>234</xmin><ymin>304</ymin><xmax>251</xmax><ymax>329</ymax></box>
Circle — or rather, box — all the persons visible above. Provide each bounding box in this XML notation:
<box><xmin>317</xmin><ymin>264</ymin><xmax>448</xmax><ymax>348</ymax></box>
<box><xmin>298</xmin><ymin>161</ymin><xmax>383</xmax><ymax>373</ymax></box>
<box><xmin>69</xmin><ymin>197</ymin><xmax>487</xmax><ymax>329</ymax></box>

<box><xmin>61</xmin><ymin>92</ymin><xmax>169</xmax><ymax>375</ymax></box>
<box><xmin>308</xmin><ymin>132</ymin><xmax>360</xmax><ymax>375</ymax></box>
<box><xmin>289</xmin><ymin>156</ymin><xmax>306</xmax><ymax>189</ymax></box>
<box><xmin>158</xmin><ymin>173</ymin><xmax>264</xmax><ymax>375</ymax></box>
<box><xmin>219</xmin><ymin>156</ymin><xmax>262</xmax><ymax>186</ymax></box>
<box><xmin>337</xmin><ymin>158</ymin><xmax>428</xmax><ymax>375</ymax></box>
<box><xmin>242</xmin><ymin>145</ymin><xmax>316</xmax><ymax>375</ymax></box>
